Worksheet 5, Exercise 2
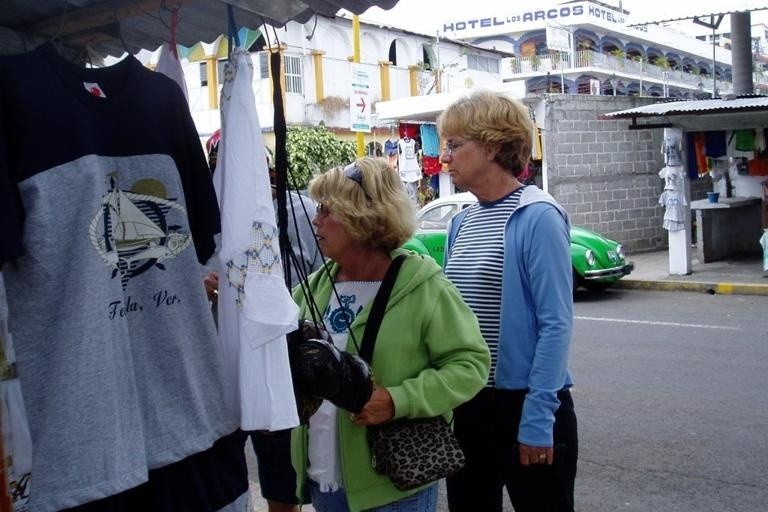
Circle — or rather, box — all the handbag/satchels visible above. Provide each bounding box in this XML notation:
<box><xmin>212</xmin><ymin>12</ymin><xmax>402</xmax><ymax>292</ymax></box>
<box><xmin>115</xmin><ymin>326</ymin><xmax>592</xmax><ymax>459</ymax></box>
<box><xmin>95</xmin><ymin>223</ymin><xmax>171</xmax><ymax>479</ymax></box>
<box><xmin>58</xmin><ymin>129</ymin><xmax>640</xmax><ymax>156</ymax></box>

<box><xmin>369</xmin><ymin>414</ymin><xmax>467</xmax><ymax>491</ymax></box>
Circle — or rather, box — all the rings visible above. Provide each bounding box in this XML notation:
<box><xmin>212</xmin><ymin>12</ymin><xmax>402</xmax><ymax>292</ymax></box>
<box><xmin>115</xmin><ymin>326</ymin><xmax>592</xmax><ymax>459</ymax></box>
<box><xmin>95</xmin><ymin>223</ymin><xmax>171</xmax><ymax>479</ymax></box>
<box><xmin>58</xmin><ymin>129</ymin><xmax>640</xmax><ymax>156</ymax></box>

<box><xmin>539</xmin><ymin>453</ymin><xmax>546</xmax><ymax>459</ymax></box>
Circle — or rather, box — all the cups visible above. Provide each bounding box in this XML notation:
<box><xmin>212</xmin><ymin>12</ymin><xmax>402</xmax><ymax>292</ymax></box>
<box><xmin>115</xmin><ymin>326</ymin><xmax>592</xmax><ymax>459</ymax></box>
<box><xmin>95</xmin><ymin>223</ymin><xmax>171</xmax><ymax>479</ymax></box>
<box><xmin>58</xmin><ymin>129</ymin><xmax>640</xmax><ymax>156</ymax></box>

<box><xmin>706</xmin><ymin>192</ymin><xmax>720</xmax><ymax>203</ymax></box>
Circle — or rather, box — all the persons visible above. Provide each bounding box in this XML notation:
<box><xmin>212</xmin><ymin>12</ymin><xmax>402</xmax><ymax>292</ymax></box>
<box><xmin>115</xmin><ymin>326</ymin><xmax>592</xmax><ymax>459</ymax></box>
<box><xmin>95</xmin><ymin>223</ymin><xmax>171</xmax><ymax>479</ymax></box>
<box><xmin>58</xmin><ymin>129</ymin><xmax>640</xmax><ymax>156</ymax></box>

<box><xmin>435</xmin><ymin>89</ymin><xmax>580</xmax><ymax>512</ymax></box>
<box><xmin>199</xmin><ymin>156</ymin><xmax>494</xmax><ymax>512</ymax></box>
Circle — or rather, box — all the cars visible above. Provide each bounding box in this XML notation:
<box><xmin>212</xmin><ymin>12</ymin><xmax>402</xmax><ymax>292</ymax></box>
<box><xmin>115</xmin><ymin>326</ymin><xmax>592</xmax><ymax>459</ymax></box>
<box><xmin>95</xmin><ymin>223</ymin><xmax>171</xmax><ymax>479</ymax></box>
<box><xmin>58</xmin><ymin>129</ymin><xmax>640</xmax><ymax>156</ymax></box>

<box><xmin>398</xmin><ymin>192</ymin><xmax>634</xmax><ymax>298</ymax></box>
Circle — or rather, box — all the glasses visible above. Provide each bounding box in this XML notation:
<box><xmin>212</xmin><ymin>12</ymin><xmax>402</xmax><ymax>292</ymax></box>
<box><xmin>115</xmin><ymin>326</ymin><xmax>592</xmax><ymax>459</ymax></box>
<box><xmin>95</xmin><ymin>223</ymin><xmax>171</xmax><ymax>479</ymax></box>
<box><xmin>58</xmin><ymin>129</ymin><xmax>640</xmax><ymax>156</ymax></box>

<box><xmin>344</xmin><ymin>161</ymin><xmax>375</xmax><ymax>206</ymax></box>
<box><xmin>444</xmin><ymin>142</ymin><xmax>465</xmax><ymax>157</ymax></box>
